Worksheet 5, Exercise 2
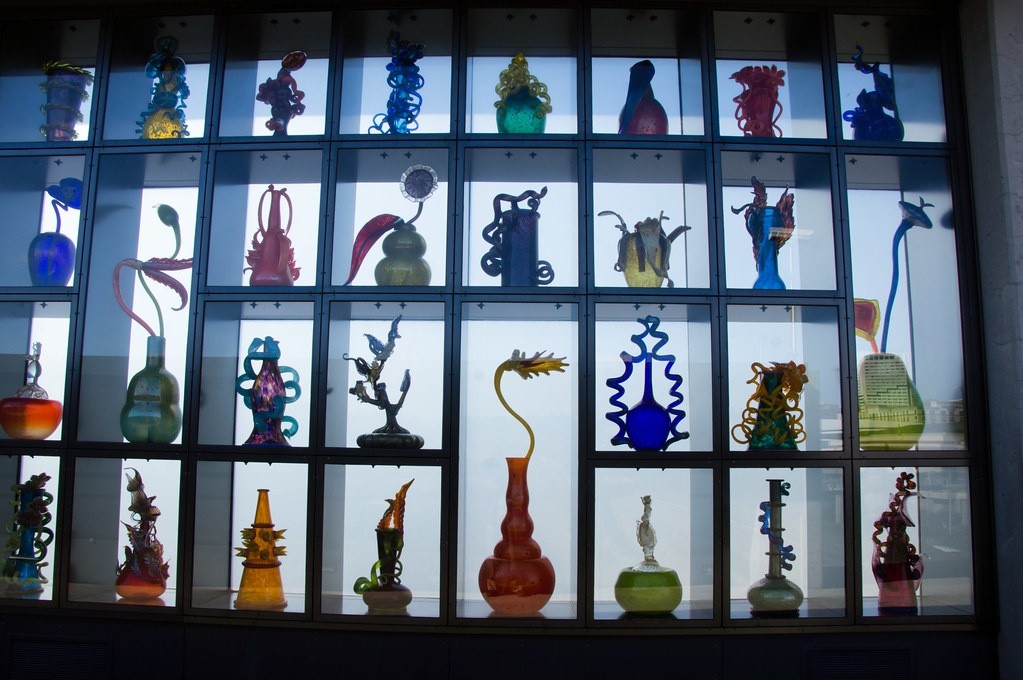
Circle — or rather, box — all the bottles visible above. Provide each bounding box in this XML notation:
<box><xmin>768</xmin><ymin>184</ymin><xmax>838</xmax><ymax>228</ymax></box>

<box><xmin>478</xmin><ymin>456</ymin><xmax>555</xmax><ymax>615</ymax></box>
<box><xmin>119</xmin><ymin>336</ymin><xmax>180</xmax><ymax>444</ymax></box>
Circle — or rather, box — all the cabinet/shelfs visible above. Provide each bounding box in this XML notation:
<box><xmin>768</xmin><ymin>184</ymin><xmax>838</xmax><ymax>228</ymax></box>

<box><xmin>1</xmin><ymin>0</ymin><xmax>1000</xmax><ymax>637</ymax></box>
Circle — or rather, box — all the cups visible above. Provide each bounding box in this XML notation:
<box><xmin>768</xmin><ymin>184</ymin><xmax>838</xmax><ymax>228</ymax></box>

<box><xmin>39</xmin><ymin>63</ymin><xmax>92</xmax><ymax>140</ymax></box>
<box><xmin>745</xmin><ymin>207</ymin><xmax>787</xmax><ymax>289</ymax></box>
